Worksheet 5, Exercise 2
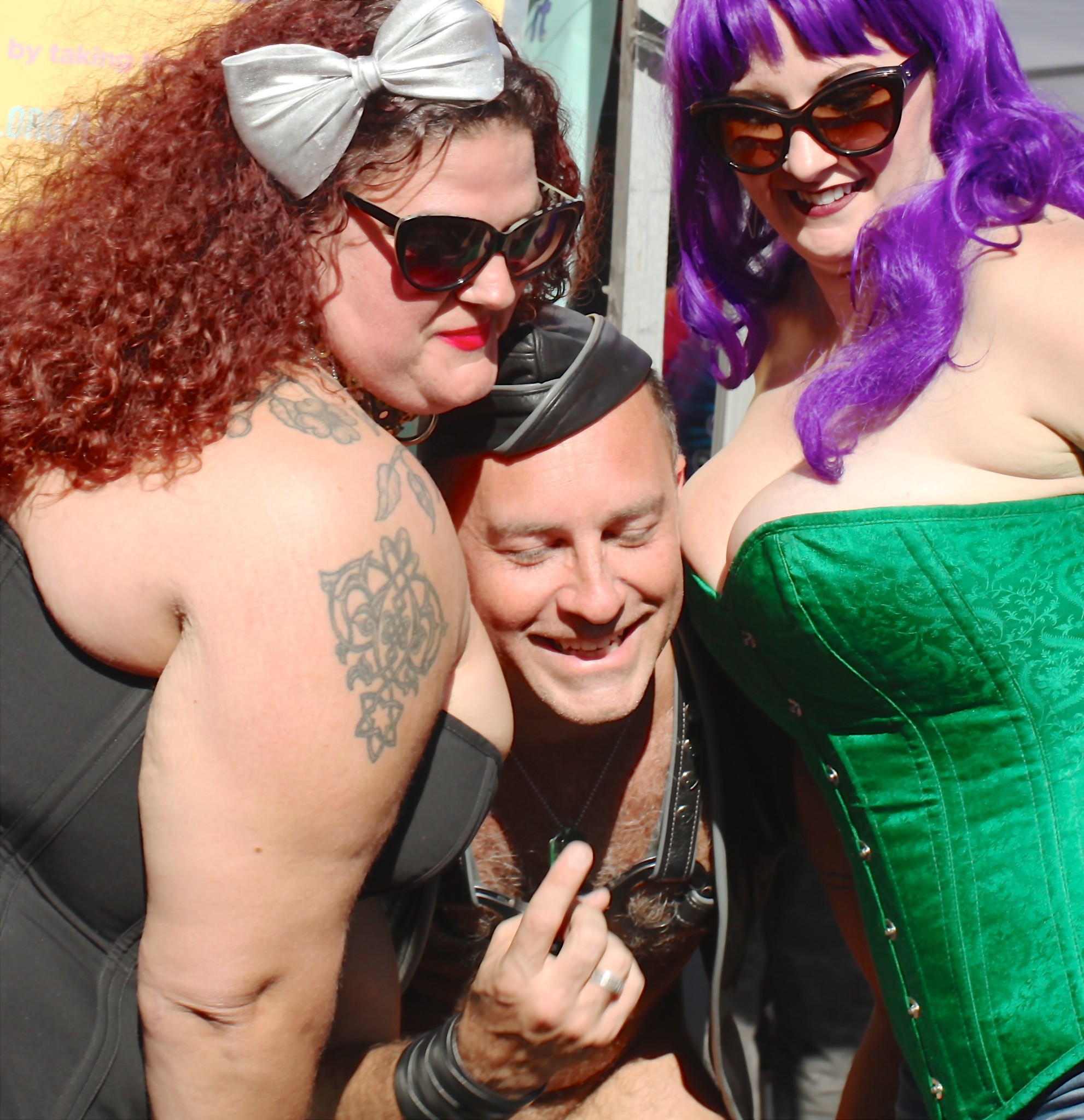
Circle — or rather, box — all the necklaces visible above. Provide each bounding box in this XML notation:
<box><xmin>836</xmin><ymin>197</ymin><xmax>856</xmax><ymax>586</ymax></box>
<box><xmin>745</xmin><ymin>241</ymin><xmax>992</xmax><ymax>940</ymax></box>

<box><xmin>495</xmin><ymin>706</ymin><xmax>631</xmax><ymax>874</ymax></box>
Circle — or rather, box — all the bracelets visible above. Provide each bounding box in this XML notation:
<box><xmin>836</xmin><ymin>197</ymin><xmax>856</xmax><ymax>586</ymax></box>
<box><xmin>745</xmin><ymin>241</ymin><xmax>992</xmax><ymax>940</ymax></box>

<box><xmin>395</xmin><ymin>1011</ymin><xmax>547</xmax><ymax>1120</ymax></box>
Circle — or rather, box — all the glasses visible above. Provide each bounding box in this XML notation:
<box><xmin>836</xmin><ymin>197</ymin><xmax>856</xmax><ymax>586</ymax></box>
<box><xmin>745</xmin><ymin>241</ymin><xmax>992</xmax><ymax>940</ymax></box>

<box><xmin>338</xmin><ymin>177</ymin><xmax>587</xmax><ymax>292</ymax></box>
<box><xmin>686</xmin><ymin>45</ymin><xmax>931</xmax><ymax>176</ymax></box>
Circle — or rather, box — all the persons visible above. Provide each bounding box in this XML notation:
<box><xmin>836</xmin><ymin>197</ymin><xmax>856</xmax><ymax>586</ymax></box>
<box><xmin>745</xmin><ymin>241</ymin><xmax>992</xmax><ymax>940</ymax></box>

<box><xmin>308</xmin><ymin>303</ymin><xmax>881</xmax><ymax>1120</ymax></box>
<box><xmin>1</xmin><ymin>0</ymin><xmax>608</xmax><ymax>1120</ymax></box>
<box><xmin>664</xmin><ymin>0</ymin><xmax>1084</xmax><ymax>1119</ymax></box>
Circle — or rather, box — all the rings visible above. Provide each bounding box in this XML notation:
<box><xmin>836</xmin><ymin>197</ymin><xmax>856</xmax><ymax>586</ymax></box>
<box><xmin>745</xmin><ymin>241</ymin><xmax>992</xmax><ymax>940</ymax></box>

<box><xmin>589</xmin><ymin>968</ymin><xmax>625</xmax><ymax>997</ymax></box>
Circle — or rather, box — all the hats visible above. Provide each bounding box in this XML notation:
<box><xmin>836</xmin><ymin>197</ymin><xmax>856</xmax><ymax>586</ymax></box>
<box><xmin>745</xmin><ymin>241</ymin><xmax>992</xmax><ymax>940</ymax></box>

<box><xmin>438</xmin><ymin>299</ymin><xmax>654</xmax><ymax>460</ymax></box>
